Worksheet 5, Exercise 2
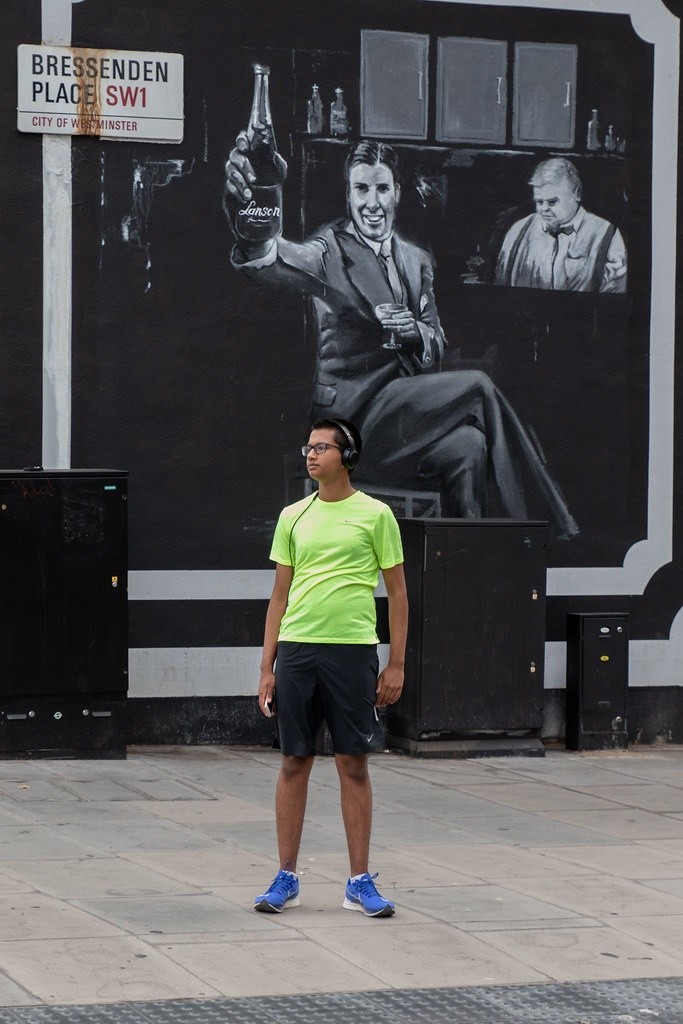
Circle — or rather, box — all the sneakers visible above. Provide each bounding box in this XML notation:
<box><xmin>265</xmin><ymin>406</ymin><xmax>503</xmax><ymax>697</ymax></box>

<box><xmin>343</xmin><ymin>872</ymin><xmax>396</xmax><ymax>917</ymax></box>
<box><xmin>253</xmin><ymin>870</ymin><xmax>300</xmax><ymax>913</ymax></box>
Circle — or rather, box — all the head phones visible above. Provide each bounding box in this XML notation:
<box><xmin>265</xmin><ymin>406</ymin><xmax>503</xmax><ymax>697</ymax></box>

<box><xmin>323</xmin><ymin>417</ymin><xmax>359</xmax><ymax>469</ymax></box>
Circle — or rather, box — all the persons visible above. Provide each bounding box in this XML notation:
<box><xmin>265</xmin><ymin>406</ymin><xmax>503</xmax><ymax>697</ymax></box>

<box><xmin>253</xmin><ymin>418</ymin><xmax>408</xmax><ymax>917</ymax></box>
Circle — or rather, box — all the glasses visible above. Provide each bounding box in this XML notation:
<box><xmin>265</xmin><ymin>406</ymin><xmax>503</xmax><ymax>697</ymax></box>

<box><xmin>301</xmin><ymin>442</ymin><xmax>343</xmax><ymax>457</ymax></box>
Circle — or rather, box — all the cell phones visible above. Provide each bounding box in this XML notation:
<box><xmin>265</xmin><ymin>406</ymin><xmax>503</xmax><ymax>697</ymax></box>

<box><xmin>264</xmin><ymin>690</ymin><xmax>275</xmax><ymax>717</ymax></box>
